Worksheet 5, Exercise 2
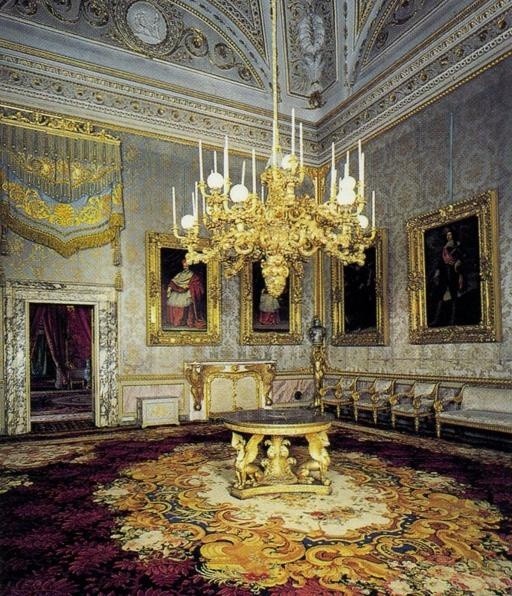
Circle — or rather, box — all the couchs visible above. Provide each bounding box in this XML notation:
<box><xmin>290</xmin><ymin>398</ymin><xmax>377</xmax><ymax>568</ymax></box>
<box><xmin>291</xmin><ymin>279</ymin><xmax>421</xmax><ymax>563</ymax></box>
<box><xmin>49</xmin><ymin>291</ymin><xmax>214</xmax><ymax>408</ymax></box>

<box><xmin>433</xmin><ymin>381</ymin><xmax>512</xmax><ymax>439</ymax></box>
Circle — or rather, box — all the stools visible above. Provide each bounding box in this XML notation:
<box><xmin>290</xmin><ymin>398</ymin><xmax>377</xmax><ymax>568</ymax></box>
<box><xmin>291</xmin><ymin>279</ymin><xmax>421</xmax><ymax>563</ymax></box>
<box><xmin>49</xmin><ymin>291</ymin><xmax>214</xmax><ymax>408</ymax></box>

<box><xmin>70</xmin><ymin>378</ymin><xmax>84</xmax><ymax>388</ymax></box>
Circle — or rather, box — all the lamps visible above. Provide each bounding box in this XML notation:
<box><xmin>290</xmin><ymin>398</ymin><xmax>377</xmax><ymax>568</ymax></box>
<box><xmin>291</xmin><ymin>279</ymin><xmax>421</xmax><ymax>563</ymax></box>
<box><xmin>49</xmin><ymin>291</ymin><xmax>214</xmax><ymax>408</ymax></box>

<box><xmin>167</xmin><ymin>0</ymin><xmax>379</xmax><ymax>301</ymax></box>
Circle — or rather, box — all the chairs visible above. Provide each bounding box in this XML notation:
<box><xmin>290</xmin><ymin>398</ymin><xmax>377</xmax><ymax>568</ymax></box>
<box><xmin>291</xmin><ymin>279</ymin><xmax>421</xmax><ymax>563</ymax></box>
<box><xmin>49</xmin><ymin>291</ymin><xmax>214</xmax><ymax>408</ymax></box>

<box><xmin>319</xmin><ymin>376</ymin><xmax>441</xmax><ymax>434</ymax></box>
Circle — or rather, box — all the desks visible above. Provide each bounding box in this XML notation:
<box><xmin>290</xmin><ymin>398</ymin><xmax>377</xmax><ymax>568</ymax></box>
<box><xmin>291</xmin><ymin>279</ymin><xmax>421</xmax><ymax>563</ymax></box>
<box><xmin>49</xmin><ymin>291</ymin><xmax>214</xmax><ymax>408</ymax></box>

<box><xmin>224</xmin><ymin>406</ymin><xmax>335</xmax><ymax>498</ymax></box>
<box><xmin>183</xmin><ymin>358</ymin><xmax>279</xmax><ymax>425</ymax></box>
<box><xmin>67</xmin><ymin>369</ymin><xmax>85</xmax><ymax>389</ymax></box>
<box><xmin>136</xmin><ymin>394</ymin><xmax>181</xmax><ymax>429</ymax></box>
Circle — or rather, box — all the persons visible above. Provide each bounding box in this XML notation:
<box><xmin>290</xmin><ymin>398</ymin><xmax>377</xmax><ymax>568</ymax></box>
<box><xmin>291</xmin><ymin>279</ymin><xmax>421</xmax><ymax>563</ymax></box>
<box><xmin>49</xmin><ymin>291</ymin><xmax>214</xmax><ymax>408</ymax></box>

<box><xmin>166</xmin><ymin>258</ymin><xmax>207</xmax><ymax>328</ymax></box>
<box><xmin>258</xmin><ymin>286</ymin><xmax>281</xmax><ymax>325</ymax></box>
<box><xmin>428</xmin><ymin>228</ymin><xmax>468</xmax><ymax>327</ymax></box>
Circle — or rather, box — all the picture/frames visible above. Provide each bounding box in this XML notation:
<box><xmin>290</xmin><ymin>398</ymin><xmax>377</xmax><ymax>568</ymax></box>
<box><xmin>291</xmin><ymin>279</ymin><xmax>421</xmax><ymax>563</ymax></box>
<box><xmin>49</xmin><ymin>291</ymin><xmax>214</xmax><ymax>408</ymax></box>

<box><xmin>240</xmin><ymin>260</ymin><xmax>305</xmax><ymax>348</ymax></box>
<box><xmin>142</xmin><ymin>231</ymin><xmax>220</xmax><ymax>349</ymax></box>
<box><xmin>328</xmin><ymin>226</ymin><xmax>392</xmax><ymax>348</ymax></box>
<box><xmin>403</xmin><ymin>189</ymin><xmax>503</xmax><ymax>348</ymax></box>
